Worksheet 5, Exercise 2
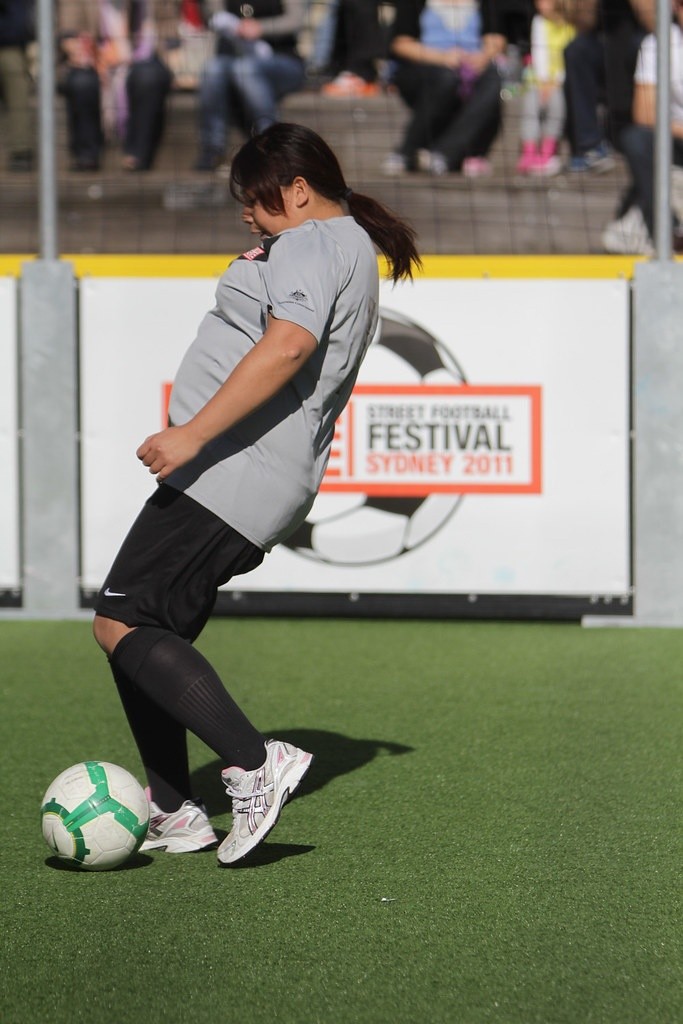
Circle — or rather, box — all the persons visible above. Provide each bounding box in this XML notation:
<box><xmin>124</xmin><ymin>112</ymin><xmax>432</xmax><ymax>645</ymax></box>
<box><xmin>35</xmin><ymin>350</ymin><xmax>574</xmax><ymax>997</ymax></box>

<box><xmin>313</xmin><ymin>0</ymin><xmax>683</xmax><ymax>254</ymax></box>
<box><xmin>55</xmin><ymin>0</ymin><xmax>311</xmax><ymax>176</ymax></box>
<box><xmin>93</xmin><ymin>121</ymin><xmax>424</xmax><ymax>868</ymax></box>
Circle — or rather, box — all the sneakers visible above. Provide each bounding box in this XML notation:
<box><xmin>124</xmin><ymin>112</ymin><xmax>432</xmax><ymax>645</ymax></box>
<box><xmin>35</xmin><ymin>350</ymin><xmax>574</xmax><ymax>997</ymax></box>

<box><xmin>138</xmin><ymin>787</ymin><xmax>218</xmax><ymax>854</ymax></box>
<box><xmin>217</xmin><ymin>739</ymin><xmax>315</xmax><ymax>864</ymax></box>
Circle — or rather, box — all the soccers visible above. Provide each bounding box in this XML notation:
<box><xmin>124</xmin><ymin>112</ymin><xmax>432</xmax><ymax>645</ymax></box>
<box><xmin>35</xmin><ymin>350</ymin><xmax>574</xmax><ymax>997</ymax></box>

<box><xmin>40</xmin><ymin>758</ymin><xmax>151</xmax><ymax>868</ymax></box>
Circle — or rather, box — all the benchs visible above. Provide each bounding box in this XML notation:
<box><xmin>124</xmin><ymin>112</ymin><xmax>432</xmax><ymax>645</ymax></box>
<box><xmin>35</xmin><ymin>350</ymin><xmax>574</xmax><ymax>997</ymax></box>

<box><xmin>27</xmin><ymin>91</ymin><xmax>523</xmax><ymax>184</ymax></box>
